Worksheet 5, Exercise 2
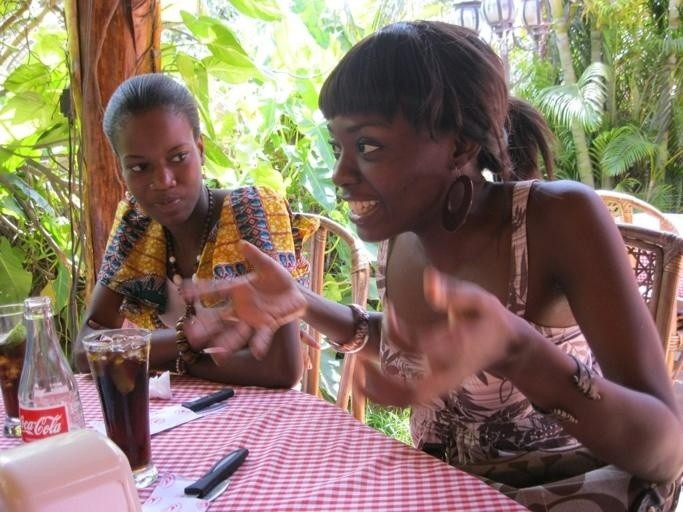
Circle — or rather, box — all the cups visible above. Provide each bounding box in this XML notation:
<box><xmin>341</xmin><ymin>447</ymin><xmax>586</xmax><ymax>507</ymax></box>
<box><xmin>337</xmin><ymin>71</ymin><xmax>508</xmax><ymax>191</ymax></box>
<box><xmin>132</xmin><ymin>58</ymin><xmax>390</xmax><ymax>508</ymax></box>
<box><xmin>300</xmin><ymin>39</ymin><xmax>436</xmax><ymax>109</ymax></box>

<box><xmin>80</xmin><ymin>328</ymin><xmax>160</xmax><ymax>488</ymax></box>
<box><xmin>0</xmin><ymin>305</ymin><xmax>32</xmax><ymax>436</ymax></box>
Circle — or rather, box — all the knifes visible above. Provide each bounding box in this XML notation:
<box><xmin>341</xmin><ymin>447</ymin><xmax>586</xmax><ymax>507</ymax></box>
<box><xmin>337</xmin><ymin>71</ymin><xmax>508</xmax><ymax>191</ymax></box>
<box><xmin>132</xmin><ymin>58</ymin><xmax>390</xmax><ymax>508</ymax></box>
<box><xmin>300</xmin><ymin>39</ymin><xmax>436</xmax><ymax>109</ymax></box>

<box><xmin>158</xmin><ymin>448</ymin><xmax>247</xmax><ymax>512</ymax></box>
<box><xmin>148</xmin><ymin>388</ymin><xmax>234</xmax><ymax>437</ymax></box>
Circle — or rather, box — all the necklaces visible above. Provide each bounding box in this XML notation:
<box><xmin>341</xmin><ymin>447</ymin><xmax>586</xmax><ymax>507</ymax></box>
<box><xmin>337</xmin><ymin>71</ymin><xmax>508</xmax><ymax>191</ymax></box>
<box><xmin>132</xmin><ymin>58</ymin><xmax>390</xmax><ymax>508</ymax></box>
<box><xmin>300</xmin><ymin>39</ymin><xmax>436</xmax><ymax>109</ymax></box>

<box><xmin>165</xmin><ymin>185</ymin><xmax>214</xmax><ymax>317</ymax></box>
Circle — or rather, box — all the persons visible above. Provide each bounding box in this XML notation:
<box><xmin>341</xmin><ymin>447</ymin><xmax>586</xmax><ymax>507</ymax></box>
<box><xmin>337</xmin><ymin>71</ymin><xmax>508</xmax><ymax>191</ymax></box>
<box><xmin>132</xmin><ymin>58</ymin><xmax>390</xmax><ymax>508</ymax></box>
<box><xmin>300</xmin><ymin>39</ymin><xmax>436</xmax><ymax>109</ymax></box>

<box><xmin>71</xmin><ymin>71</ymin><xmax>321</xmax><ymax>391</ymax></box>
<box><xmin>179</xmin><ymin>19</ymin><xmax>683</xmax><ymax>511</ymax></box>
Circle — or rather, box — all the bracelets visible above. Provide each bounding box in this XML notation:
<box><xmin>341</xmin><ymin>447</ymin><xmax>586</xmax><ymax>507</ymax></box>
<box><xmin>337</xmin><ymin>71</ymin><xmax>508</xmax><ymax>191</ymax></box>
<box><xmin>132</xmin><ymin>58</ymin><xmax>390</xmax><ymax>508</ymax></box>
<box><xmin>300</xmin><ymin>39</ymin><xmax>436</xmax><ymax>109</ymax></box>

<box><xmin>326</xmin><ymin>302</ymin><xmax>371</xmax><ymax>355</ymax></box>
<box><xmin>530</xmin><ymin>353</ymin><xmax>600</xmax><ymax>428</ymax></box>
<box><xmin>174</xmin><ymin>315</ymin><xmax>201</xmax><ymax>366</ymax></box>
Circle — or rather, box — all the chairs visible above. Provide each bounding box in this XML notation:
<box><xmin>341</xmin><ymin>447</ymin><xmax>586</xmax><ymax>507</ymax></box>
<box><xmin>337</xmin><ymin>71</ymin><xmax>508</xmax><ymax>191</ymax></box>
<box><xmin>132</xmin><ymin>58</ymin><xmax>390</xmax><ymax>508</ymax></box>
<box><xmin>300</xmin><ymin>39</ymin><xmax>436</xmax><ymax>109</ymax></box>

<box><xmin>595</xmin><ymin>188</ymin><xmax>678</xmax><ymax>233</ymax></box>
<box><xmin>613</xmin><ymin>222</ymin><xmax>679</xmax><ymax>364</ymax></box>
<box><xmin>288</xmin><ymin>211</ymin><xmax>369</xmax><ymax>423</ymax></box>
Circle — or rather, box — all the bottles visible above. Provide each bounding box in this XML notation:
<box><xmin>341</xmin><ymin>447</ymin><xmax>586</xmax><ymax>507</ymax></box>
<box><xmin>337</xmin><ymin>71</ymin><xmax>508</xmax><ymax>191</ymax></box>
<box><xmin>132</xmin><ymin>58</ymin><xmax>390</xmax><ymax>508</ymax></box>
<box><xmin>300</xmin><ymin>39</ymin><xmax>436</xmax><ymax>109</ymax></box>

<box><xmin>18</xmin><ymin>296</ymin><xmax>86</xmax><ymax>442</ymax></box>
